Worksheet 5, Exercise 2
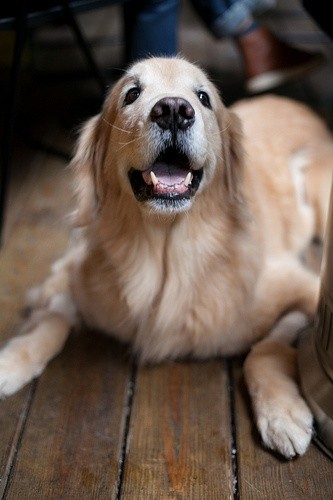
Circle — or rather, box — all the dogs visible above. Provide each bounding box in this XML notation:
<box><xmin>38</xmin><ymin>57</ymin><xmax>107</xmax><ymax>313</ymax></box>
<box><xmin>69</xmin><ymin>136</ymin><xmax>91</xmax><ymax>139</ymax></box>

<box><xmin>0</xmin><ymin>53</ymin><xmax>333</xmax><ymax>462</ymax></box>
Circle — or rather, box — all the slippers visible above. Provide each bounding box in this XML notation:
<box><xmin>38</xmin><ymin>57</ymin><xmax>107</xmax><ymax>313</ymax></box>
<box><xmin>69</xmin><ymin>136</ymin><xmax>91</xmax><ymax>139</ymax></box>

<box><xmin>245</xmin><ymin>49</ymin><xmax>327</xmax><ymax>97</ymax></box>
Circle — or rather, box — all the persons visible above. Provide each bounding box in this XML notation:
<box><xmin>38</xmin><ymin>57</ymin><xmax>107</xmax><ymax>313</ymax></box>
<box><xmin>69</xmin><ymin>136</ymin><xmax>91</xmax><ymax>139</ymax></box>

<box><xmin>119</xmin><ymin>0</ymin><xmax>328</xmax><ymax>95</ymax></box>
